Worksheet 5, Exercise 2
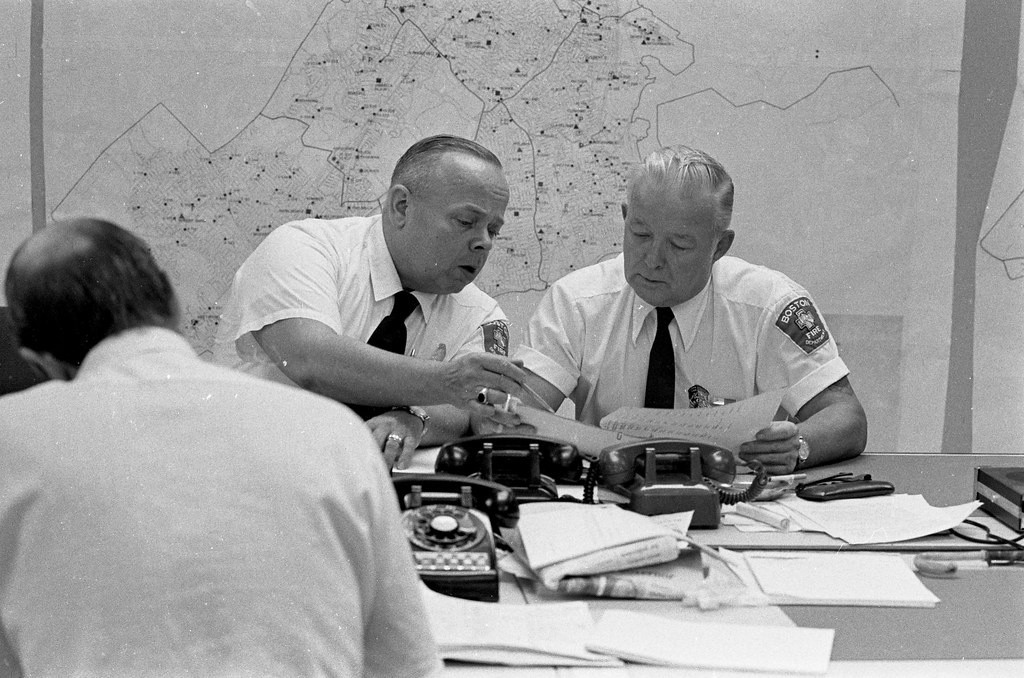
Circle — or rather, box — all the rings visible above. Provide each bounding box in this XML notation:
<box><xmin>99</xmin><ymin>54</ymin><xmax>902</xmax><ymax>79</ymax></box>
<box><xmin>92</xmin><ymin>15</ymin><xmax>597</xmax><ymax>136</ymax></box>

<box><xmin>388</xmin><ymin>433</ymin><xmax>401</xmax><ymax>443</ymax></box>
<box><xmin>478</xmin><ymin>387</ymin><xmax>487</xmax><ymax>403</ymax></box>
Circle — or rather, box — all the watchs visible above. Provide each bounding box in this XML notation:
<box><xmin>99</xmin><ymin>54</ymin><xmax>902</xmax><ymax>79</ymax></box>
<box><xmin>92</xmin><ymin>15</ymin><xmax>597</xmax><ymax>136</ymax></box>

<box><xmin>799</xmin><ymin>436</ymin><xmax>810</xmax><ymax>465</ymax></box>
<box><xmin>392</xmin><ymin>406</ymin><xmax>430</xmax><ymax>436</ymax></box>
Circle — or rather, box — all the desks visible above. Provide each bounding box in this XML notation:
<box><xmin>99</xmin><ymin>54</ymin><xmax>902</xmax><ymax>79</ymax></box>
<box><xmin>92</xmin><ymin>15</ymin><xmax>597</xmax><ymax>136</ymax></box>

<box><xmin>443</xmin><ymin>451</ymin><xmax>1024</xmax><ymax>677</ymax></box>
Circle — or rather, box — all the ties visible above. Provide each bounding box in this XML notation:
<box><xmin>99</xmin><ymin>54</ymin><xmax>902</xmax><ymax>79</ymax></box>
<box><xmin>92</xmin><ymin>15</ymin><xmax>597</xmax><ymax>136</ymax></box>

<box><xmin>641</xmin><ymin>306</ymin><xmax>676</xmax><ymax>409</ymax></box>
<box><xmin>340</xmin><ymin>293</ymin><xmax>420</xmax><ymax>421</ymax></box>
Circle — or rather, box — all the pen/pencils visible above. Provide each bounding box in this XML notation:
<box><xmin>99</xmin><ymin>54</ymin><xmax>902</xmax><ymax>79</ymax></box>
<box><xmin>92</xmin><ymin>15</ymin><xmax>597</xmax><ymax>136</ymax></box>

<box><xmin>497</xmin><ymin>394</ymin><xmax>511</xmax><ymax>437</ymax></box>
<box><xmin>517</xmin><ymin>379</ymin><xmax>556</xmax><ymax>414</ymax></box>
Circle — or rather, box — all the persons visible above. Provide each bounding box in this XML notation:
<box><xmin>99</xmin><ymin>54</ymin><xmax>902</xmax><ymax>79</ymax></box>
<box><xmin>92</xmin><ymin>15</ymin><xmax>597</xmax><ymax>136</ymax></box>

<box><xmin>0</xmin><ymin>216</ymin><xmax>446</xmax><ymax>677</ymax></box>
<box><xmin>470</xmin><ymin>145</ymin><xmax>868</xmax><ymax>475</ymax></box>
<box><xmin>212</xmin><ymin>136</ymin><xmax>526</xmax><ymax>475</ymax></box>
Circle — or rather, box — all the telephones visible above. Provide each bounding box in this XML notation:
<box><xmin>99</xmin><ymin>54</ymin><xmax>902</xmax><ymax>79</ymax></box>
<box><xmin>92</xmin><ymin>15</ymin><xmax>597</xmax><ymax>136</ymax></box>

<box><xmin>432</xmin><ymin>432</ymin><xmax>582</xmax><ymax>524</ymax></box>
<box><xmin>390</xmin><ymin>472</ymin><xmax>520</xmax><ymax>603</ymax></box>
<box><xmin>584</xmin><ymin>437</ymin><xmax>770</xmax><ymax>529</ymax></box>
